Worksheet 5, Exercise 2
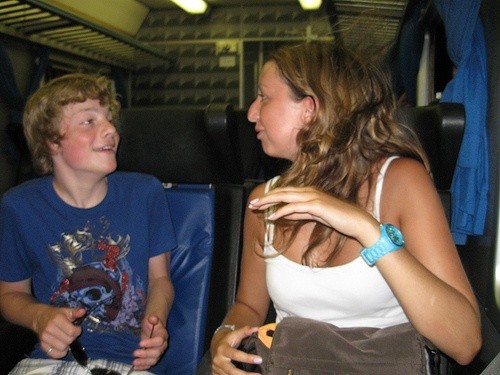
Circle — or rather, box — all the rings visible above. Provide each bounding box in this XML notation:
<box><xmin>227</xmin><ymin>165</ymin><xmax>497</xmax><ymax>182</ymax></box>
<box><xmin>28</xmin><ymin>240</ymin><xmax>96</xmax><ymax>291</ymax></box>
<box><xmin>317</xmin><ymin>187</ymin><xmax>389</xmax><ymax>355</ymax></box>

<box><xmin>45</xmin><ymin>347</ymin><xmax>53</xmax><ymax>355</ymax></box>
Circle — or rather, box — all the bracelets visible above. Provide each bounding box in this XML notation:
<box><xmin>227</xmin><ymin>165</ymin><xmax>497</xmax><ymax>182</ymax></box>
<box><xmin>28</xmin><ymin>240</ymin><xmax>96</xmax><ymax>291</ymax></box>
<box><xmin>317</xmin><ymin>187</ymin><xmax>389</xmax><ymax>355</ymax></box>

<box><xmin>212</xmin><ymin>324</ymin><xmax>236</xmax><ymax>336</ymax></box>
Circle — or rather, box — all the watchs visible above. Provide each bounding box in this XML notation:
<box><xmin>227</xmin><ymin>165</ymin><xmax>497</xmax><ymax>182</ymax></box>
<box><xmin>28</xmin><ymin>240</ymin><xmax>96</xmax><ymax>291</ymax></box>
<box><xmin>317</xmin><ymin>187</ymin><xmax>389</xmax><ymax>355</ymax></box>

<box><xmin>359</xmin><ymin>222</ymin><xmax>406</xmax><ymax>268</ymax></box>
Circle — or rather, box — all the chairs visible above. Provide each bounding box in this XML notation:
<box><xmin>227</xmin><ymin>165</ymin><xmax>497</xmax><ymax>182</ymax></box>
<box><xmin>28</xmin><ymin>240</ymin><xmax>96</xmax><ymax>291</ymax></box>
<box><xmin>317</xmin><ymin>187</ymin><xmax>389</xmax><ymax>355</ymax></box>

<box><xmin>103</xmin><ymin>103</ymin><xmax>468</xmax><ymax>375</ymax></box>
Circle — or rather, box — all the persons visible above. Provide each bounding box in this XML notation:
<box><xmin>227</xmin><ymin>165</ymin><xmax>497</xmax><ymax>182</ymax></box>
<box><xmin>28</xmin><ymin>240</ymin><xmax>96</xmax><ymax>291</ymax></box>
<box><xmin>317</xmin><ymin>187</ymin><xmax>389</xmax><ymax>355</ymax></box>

<box><xmin>0</xmin><ymin>73</ymin><xmax>178</xmax><ymax>375</ymax></box>
<box><xmin>210</xmin><ymin>40</ymin><xmax>482</xmax><ymax>375</ymax></box>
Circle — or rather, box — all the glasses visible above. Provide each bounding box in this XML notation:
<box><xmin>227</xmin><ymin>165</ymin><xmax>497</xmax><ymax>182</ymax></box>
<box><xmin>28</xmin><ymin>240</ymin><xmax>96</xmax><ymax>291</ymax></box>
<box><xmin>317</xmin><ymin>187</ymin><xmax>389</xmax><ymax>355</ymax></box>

<box><xmin>70</xmin><ymin>295</ymin><xmax>155</xmax><ymax>375</ymax></box>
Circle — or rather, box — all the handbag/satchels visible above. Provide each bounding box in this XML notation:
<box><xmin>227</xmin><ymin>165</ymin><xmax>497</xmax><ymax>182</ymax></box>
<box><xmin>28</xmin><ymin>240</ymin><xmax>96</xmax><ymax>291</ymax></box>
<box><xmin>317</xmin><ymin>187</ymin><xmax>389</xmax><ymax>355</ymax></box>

<box><xmin>240</xmin><ymin>317</ymin><xmax>431</xmax><ymax>375</ymax></box>
